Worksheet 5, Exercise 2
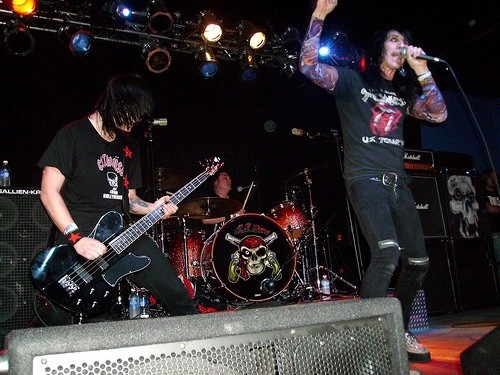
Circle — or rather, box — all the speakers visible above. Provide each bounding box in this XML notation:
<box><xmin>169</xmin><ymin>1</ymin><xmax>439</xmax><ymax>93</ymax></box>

<box><xmin>335</xmin><ymin>176</ymin><xmax>459</xmax><ymax>320</ymax></box>
<box><xmin>5</xmin><ymin>299</ymin><xmax>411</xmax><ymax>375</ymax></box>
<box><xmin>0</xmin><ymin>187</ymin><xmax>75</xmax><ymax>352</ymax></box>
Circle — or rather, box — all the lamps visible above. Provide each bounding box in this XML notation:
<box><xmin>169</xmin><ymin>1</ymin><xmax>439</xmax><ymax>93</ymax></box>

<box><xmin>0</xmin><ymin>0</ymin><xmax>331</xmax><ymax>85</ymax></box>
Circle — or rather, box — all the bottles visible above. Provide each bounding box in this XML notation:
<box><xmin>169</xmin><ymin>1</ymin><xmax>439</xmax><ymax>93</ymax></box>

<box><xmin>0</xmin><ymin>161</ymin><xmax>10</xmax><ymax>186</ymax></box>
<box><xmin>129</xmin><ymin>288</ymin><xmax>140</xmax><ymax>318</ymax></box>
<box><xmin>321</xmin><ymin>275</ymin><xmax>331</xmax><ymax>301</ymax></box>
<box><xmin>139</xmin><ymin>288</ymin><xmax>150</xmax><ymax>318</ymax></box>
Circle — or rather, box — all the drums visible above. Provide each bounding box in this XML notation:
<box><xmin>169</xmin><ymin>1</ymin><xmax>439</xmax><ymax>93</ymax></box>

<box><xmin>269</xmin><ymin>201</ymin><xmax>311</xmax><ymax>239</ymax></box>
<box><xmin>160</xmin><ymin>230</ymin><xmax>206</xmax><ymax>279</ymax></box>
<box><xmin>200</xmin><ymin>213</ymin><xmax>297</xmax><ymax>302</ymax></box>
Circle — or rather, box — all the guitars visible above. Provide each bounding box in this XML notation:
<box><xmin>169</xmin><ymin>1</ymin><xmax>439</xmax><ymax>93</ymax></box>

<box><xmin>29</xmin><ymin>156</ymin><xmax>224</xmax><ymax>318</ymax></box>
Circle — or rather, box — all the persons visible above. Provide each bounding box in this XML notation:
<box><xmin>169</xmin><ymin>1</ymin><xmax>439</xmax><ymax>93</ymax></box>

<box><xmin>298</xmin><ymin>0</ymin><xmax>448</xmax><ymax>361</ymax></box>
<box><xmin>200</xmin><ymin>169</ymin><xmax>244</xmax><ymax>239</ymax></box>
<box><xmin>37</xmin><ymin>73</ymin><xmax>198</xmax><ymax>327</ymax></box>
<box><xmin>482</xmin><ymin>167</ymin><xmax>500</xmax><ymax>274</ymax></box>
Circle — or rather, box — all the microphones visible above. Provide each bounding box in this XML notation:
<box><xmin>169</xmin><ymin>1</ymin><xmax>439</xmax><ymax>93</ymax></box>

<box><xmin>400</xmin><ymin>47</ymin><xmax>447</xmax><ymax>65</ymax></box>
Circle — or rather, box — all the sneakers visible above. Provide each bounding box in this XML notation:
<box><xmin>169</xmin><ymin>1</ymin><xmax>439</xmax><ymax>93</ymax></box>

<box><xmin>404</xmin><ymin>331</ymin><xmax>431</xmax><ymax>361</ymax></box>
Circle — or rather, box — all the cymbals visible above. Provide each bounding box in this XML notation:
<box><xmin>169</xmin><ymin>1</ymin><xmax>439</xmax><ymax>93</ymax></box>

<box><xmin>283</xmin><ymin>165</ymin><xmax>330</xmax><ymax>193</ymax></box>
<box><xmin>142</xmin><ymin>167</ymin><xmax>187</xmax><ymax>189</ymax></box>
<box><xmin>178</xmin><ymin>197</ymin><xmax>242</xmax><ymax>218</ymax></box>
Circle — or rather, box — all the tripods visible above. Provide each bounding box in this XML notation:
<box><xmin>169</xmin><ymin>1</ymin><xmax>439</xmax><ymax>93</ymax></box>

<box><xmin>291</xmin><ymin>177</ymin><xmax>357</xmax><ymax>297</ymax></box>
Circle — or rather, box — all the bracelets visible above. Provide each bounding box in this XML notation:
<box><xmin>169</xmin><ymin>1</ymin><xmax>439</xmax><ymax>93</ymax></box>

<box><xmin>419</xmin><ymin>71</ymin><xmax>431</xmax><ymax>81</ymax></box>
<box><xmin>62</xmin><ymin>223</ymin><xmax>77</xmax><ymax>234</ymax></box>
<box><xmin>224</xmin><ymin>214</ymin><xmax>231</xmax><ymax>222</ymax></box>
<box><xmin>65</xmin><ymin>229</ymin><xmax>86</xmax><ymax>244</ymax></box>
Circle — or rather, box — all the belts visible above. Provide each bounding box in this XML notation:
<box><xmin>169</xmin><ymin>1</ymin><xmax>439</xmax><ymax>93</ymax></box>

<box><xmin>369</xmin><ymin>172</ymin><xmax>402</xmax><ymax>186</ymax></box>
